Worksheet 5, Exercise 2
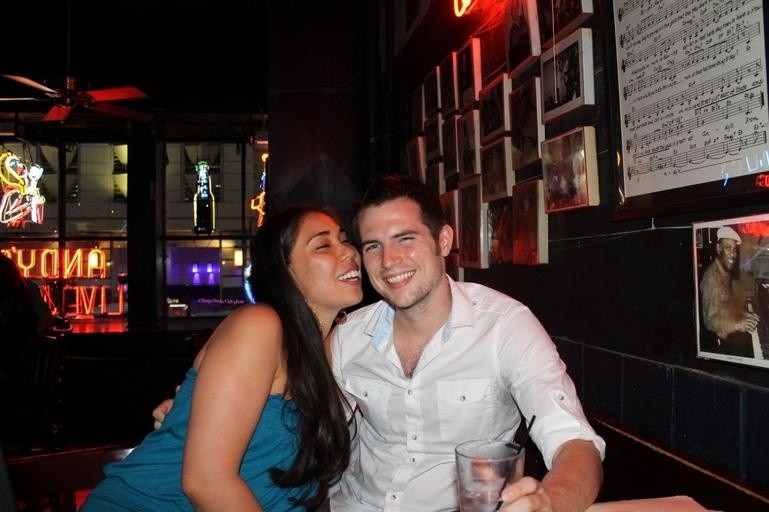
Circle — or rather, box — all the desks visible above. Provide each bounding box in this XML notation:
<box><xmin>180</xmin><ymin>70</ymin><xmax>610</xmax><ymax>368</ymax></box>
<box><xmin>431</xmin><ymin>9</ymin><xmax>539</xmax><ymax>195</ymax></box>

<box><xmin>586</xmin><ymin>496</ymin><xmax>713</xmax><ymax>512</ymax></box>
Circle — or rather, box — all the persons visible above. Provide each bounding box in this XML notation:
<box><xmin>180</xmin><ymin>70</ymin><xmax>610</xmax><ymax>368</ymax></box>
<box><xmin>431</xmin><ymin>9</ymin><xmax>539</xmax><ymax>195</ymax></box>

<box><xmin>78</xmin><ymin>202</ymin><xmax>368</xmax><ymax>512</ymax></box>
<box><xmin>698</xmin><ymin>226</ymin><xmax>761</xmax><ymax>360</ymax></box>
<box><xmin>150</xmin><ymin>174</ymin><xmax>608</xmax><ymax>512</ymax></box>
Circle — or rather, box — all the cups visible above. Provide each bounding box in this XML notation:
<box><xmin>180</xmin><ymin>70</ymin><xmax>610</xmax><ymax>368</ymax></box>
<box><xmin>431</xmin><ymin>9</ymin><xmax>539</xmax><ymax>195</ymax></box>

<box><xmin>454</xmin><ymin>439</ymin><xmax>526</xmax><ymax>512</ymax></box>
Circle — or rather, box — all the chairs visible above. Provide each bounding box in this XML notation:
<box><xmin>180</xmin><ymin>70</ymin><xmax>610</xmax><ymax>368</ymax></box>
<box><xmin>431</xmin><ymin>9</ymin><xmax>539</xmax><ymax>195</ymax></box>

<box><xmin>0</xmin><ymin>333</ymin><xmax>58</xmax><ymax>435</ymax></box>
<box><xmin>9</xmin><ymin>440</ymin><xmax>142</xmax><ymax>511</ymax></box>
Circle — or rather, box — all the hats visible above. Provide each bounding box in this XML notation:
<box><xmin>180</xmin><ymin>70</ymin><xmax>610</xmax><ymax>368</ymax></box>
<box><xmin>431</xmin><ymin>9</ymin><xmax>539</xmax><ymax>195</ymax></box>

<box><xmin>717</xmin><ymin>226</ymin><xmax>742</xmax><ymax>244</ymax></box>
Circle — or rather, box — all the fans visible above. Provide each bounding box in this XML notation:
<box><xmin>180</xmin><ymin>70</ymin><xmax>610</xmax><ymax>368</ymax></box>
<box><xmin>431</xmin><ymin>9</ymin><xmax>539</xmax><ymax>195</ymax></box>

<box><xmin>0</xmin><ymin>71</ymin><xmax>153</xmax><ymax>126</ymax></box>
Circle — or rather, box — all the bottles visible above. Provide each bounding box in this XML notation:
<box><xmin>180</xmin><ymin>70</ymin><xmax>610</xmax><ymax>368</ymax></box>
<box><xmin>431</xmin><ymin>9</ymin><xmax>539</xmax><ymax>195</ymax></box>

<box><xmin>192</xmin><ymin>160</ymin><xmax>215</xmax><ymax>235</ymax></box>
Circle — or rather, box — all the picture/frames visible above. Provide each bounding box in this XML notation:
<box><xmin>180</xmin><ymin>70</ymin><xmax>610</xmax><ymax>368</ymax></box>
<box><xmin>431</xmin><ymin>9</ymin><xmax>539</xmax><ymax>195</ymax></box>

<box><xmin>508</xmin><ymin>77</ymin><xmax>540</xmax><ymax>171</ymax></box>
<box><xmin>503</xmin><ymin>0</ymin><xmax>539</xmax><ymax>82</ymax></box>
<box><xmin>692</xmin><ymin>213</ymin><xmax>768</xmax><ymax>371</ymax></box>
<box><xmin>536</xmin><ymin>0</ymin><xmax>593</xmax><ymax>52</ymax></box>
<box><xmin>440</xmin><ymin>189</ymin><xmax>459</xmax><ymax>251</ymax></box>
<box><xmin>404</xmin><ymin>136</ymin><xmax>427</xmax><ymax>184</ymax></box>
<box><xmin>457</xmin><ymin>38</ymin><xmax>483</xmax><ymax>113</ymax></box>
<box><xmin>422</xmin><ymin>65</ymin><xmax>442</xmax><ymax>118</ymax></box>
<box><xmin>480</xmin><ymin>137</ymin><xmax>516</xmax><ymax>203</ymax></box>
<box><xmin>424</xmin><ymin>163</ymin><xmax>445</xmax><ymax>196</ymax></box>
<box><xmin>512</xmin><ymin>180</ymin><xmax>549</xmax><ymax>266</ymax></box>
<box><xmin>541</xmin><ymin>26</ymin><xmax>594</xmax><ymax>125</ymax></box>
<box><xmin>541</xmin><ymin>125</ymin><xmax>600</xmax><ymax>213</ymax></box>
<box><xmin>442</xmin><ymin>114</ymin><xmax>462</xmax><ymax>180</ymax></box>
<box><xmin>441</xmin><ymin>52</ymin><xmax>458</xmax><ymax>118</ymax></box>
<box><xmin>478</xmin><ymin>73</ymin><xmax>511</xmax><ymax>141</ymax></box>
<box><xmin>457</xmin><ymin>176</ymin><xmax>490</xmax><ymax>270</ymax></box>
<box><xmin>424</xmin><ymin>112</ymin><xmax>444</xmax><ymax>162</ymax></box>
<box><xmin>457</xmin><ymin>109</ymin><xmax>479</xmax><ymax>189</ymax></box>
<box><xmin>410</xmin><ymin>84</ymin><xmax>424</xmax><ymax>136</ymax></box>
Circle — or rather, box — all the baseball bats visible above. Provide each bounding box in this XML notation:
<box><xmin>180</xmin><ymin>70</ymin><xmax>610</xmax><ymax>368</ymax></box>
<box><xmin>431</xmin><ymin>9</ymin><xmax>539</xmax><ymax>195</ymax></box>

<box><xmin>746</xmin><ymin>298</ymin><xmax>763</xmax><ymax>358</ymax></box>
